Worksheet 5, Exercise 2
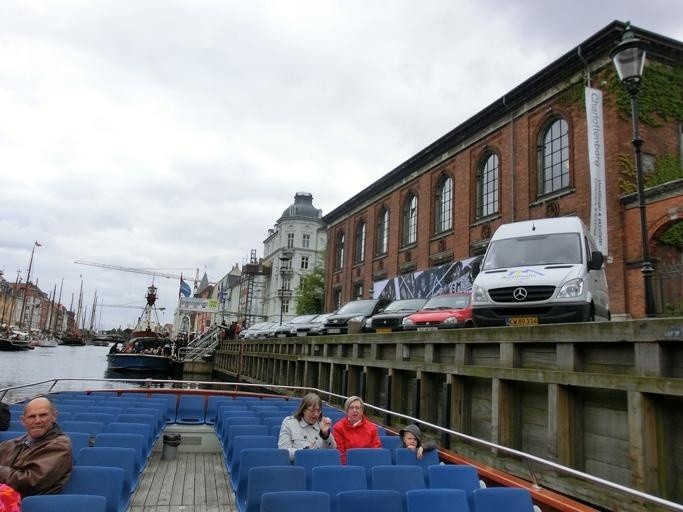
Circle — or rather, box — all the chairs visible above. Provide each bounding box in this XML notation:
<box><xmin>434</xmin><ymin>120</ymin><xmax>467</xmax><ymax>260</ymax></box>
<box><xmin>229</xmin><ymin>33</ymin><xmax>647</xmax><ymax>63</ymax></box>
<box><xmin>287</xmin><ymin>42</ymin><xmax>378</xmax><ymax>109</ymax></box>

<box><xmin>0</xmin><ymin>389</ymin><xmax>177</xmax><ymax>511</ymax></box>
<box><xmin>175</xmin><ymin>393</ymin><xmax>207</xmax><ymax>425</ymax></box>
<box><xmin>203</xmin><ymin>394</ymin><xmax>541</xmax><ymax>512</ymax></box>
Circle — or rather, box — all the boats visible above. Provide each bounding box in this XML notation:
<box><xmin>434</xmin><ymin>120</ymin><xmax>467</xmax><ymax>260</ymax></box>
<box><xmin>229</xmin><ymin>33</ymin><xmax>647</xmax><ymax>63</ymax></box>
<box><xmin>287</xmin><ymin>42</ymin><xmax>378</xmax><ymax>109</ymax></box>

<box><xmin>105</xmin><ymin>271</ymin><xmax>176</xmax><ymax>373</ymax></box>
<box><xmin>0</xmin><ymin>378</ymin><xmax>680</xmax><ymax>510</ymax></box>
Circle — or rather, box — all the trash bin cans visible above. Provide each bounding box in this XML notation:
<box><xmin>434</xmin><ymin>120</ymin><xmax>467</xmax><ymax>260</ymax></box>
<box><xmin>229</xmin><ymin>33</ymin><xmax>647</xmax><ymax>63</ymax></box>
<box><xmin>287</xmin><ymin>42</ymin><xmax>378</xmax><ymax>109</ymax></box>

<box><xmin>161</xmin><ymin>434</ymin><xmax>181</xmax><ymax>460</ymax></box>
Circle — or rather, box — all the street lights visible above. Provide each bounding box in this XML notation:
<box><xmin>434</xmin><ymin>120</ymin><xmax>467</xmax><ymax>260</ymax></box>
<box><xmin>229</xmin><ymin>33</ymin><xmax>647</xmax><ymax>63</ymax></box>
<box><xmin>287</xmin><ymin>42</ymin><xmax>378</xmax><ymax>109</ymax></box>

<box><xmin>278</xmin><ymin>250</ymin><xmax>289</xmax><ymax>326</ymax></box>
<box><xmin>606</xmin><ymin>19</ymin><xmax>659</xmax><ymax>319</ymax></box>
<box><xmin>220</xmin><ymin>292</ymin><xmax>230</xmax><ymax>324</ymax></box>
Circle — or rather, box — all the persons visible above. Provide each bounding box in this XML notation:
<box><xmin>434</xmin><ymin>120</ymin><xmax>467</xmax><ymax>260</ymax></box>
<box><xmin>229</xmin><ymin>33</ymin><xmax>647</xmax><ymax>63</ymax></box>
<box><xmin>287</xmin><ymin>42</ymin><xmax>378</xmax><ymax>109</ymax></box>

<box><xmin>277</xmin><ymin>393</ymin><xmax>336</xmax><ymax>463</ymax></box>
<box><xmin>0</xmin><ymin>397</ymin><xmax>73</xmax><ymax>501</ymax></box>
<box><xmin>114</xmin><ymin>320</ymin><xmax>246</xmax><ymax>358</ymax></box>
<box><xmin>332</xmin><ymin>396</ymin><xmax>383</xmax><ymax>465</ymax></box>
<box><xmin>395</xmin><ymin>424</ymin><xmax>437</xmax><ymax>461</ymax></box>
<box><xmin>414</xmin><ymin>262</ymin><xmax>463</xmax><ymax>300</ymax></box>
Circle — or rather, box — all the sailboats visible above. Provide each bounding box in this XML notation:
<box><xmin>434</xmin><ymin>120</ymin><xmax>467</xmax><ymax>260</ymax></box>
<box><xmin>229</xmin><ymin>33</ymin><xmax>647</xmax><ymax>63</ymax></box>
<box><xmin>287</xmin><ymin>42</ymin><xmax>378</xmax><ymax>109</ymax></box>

<box><xmin>0</xmin><ymin>241</ymin><xmax>109</xmax><ymax>349</ymax></box>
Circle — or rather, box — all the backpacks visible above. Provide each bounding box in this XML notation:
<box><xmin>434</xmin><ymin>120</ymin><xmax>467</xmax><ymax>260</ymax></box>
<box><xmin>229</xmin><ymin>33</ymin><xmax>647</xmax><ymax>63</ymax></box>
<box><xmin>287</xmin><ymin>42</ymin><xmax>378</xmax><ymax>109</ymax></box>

<box><xmin>0</xmin><ymin>401</ymin><xmax>10</xmax><ymax>431</ymax></box>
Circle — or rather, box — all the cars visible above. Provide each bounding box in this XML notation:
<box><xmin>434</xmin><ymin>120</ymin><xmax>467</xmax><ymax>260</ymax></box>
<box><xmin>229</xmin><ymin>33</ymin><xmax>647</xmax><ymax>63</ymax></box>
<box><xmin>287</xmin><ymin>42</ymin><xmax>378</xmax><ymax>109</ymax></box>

<box><xmin>239</xmin><ymin>293</ymin><xmax>472</xmax><ymax>339</ymax></box>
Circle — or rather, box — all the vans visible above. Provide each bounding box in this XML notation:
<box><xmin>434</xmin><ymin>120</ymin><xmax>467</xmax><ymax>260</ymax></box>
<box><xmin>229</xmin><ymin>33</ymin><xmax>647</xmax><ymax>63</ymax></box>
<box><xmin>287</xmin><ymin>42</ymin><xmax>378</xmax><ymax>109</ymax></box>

<box><xmin>470</xmin><ymin>215</ymin><xmax>611</xmax><ymax>325</ymax></box>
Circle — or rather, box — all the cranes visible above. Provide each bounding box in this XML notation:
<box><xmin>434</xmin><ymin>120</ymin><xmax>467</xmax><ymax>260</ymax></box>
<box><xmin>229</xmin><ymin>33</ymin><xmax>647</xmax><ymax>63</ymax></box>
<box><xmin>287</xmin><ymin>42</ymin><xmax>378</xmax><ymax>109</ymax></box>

<box><xmin>74</xmin><ymin>256</ymin><xmax>218</xmax><ymax>292</ymax></box>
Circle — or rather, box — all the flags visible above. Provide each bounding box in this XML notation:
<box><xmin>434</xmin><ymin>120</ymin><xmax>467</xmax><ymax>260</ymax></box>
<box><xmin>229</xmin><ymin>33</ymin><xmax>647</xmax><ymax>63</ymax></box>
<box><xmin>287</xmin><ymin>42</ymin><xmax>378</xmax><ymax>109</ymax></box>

<box><xmin>180</xmin><ymin>280</ymin><xmax>190</xmax><ymax>297</ymax></box>
